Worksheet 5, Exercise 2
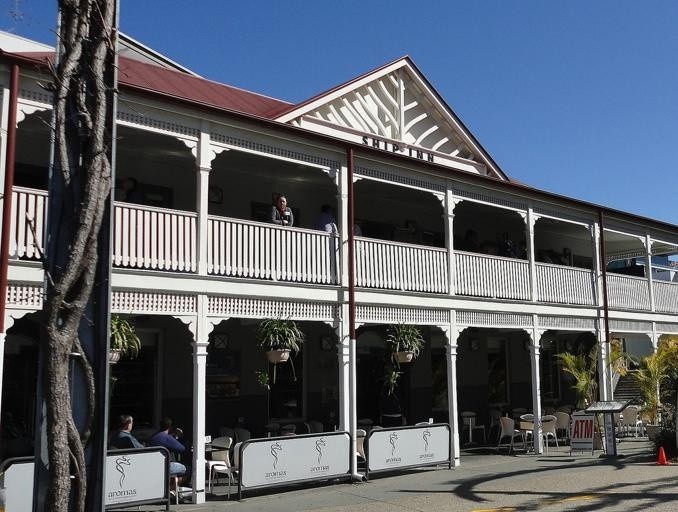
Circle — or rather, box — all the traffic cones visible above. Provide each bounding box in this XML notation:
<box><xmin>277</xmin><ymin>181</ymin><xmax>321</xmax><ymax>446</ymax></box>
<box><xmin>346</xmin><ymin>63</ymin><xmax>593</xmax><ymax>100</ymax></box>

<box><xmin>657</xmin><ymin>446</ymin><xmax>669</xmax><ymax>466</ymax></box>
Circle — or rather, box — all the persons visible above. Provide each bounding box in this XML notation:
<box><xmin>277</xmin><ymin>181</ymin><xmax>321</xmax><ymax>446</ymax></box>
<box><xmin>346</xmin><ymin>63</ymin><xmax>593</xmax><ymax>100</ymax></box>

<box><xmin>270</xmin><ymin>195</ymin><xmax>293</xmax><ymax>227</ymax></box>
<box><xmin>117</xmin><ymin>414</ymin><xmax>144</xmax><ymax>449</ymax></box>
<box><xmin>314</xmin><ymin>204</ymin><xmax>337</xmax><ymax>230</ymax></box>
<box><xmin>147</xmin><ymin>418</ymin><xmax>187</xmax><ymax>498</ymax></box>
<box><xmin>118</xmin><ymin>177</ymin><xmax>147</xmax><ymax>205</ymax></box>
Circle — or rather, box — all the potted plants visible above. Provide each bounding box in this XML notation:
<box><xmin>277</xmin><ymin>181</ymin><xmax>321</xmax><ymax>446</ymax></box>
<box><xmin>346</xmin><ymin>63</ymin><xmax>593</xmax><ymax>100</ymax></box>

<box><xmin>108</xmin><ymin>315</ymin><xmax>143</xmax><ymax>364</ymax></box>
<box><xmin>380</xmin><ymin>323</ymin><xmax>428</xmax><ymax>398</ymax></box>
<box><xmin>256</xmin><ymin>314</ymin><xmax>304</xmax><ymax>365</ymax></box>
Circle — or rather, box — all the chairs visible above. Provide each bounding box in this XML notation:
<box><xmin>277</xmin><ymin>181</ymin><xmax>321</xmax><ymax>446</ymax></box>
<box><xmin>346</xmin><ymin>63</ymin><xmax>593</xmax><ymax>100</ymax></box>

<box><xmin>110</xmin><ymin>412</ymin><xmax>434</xmax><ymax>507</ymax></box>
<box><xmin>459</xmin><ymin>403</ymin><xmax>645</xmax><ymax>457</ymax></box>
